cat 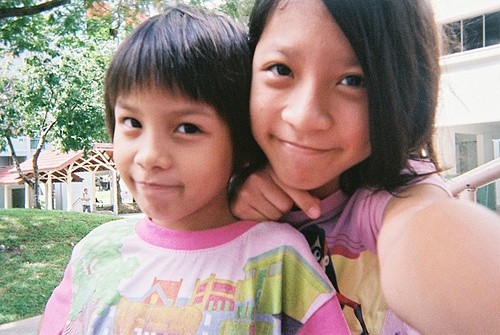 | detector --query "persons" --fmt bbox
[81,188,92,212]
[36,8,351,335]
[229,0,500,334]
